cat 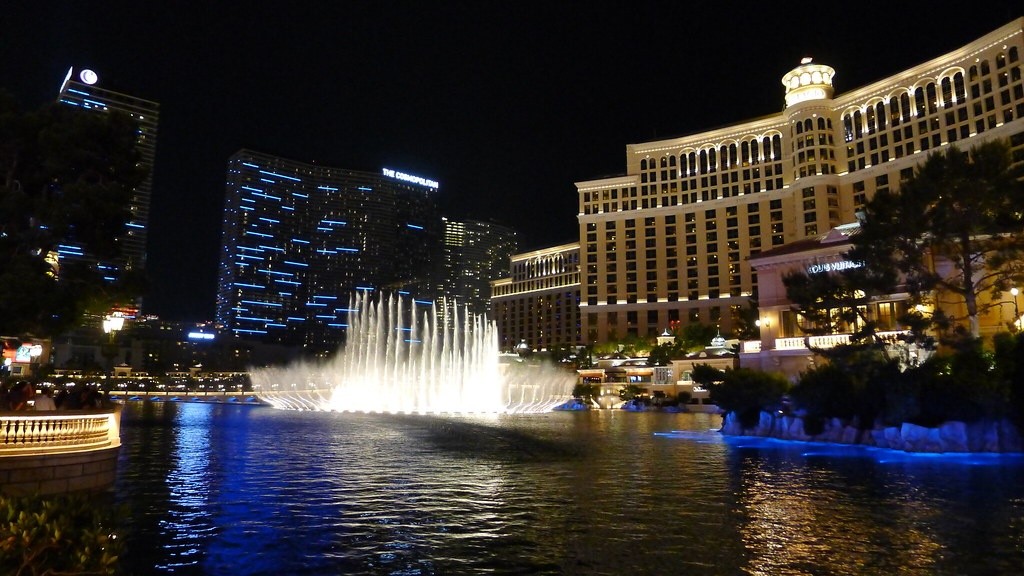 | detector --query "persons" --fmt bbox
[7,379,56,411]
